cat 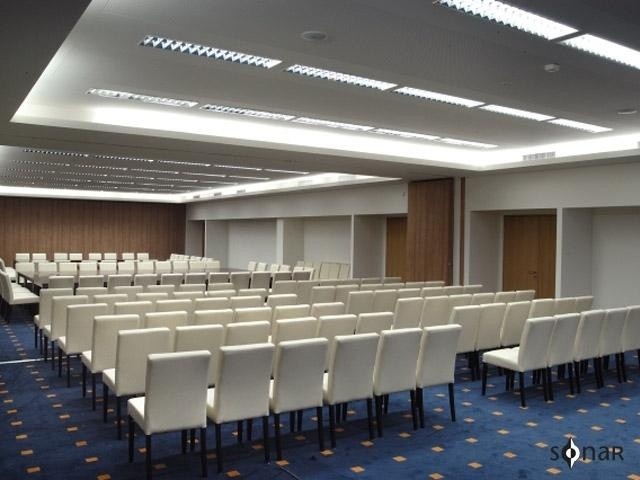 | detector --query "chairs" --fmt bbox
[32,273,640,479]
[0,249,318,324]
